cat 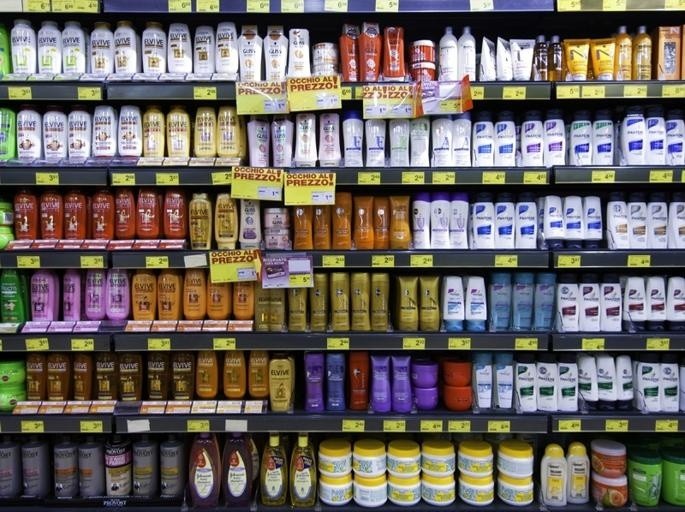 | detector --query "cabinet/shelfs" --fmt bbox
[0,1,684,512]
